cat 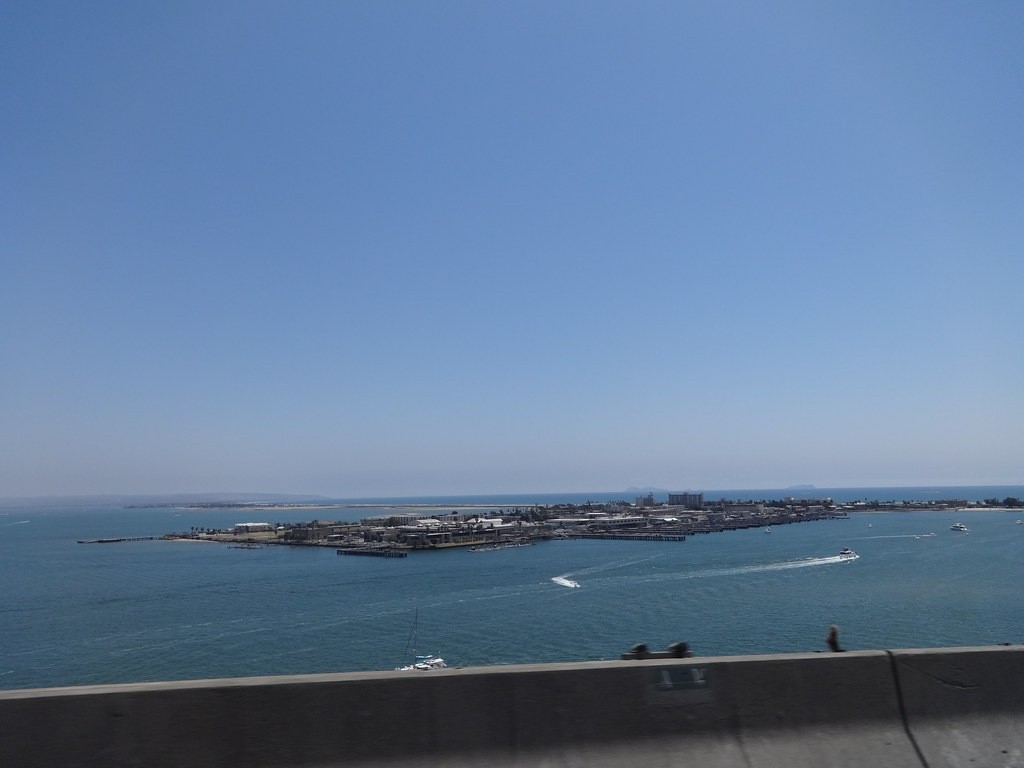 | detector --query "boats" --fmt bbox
[840,549,856,559]
[950,521,969,532]
